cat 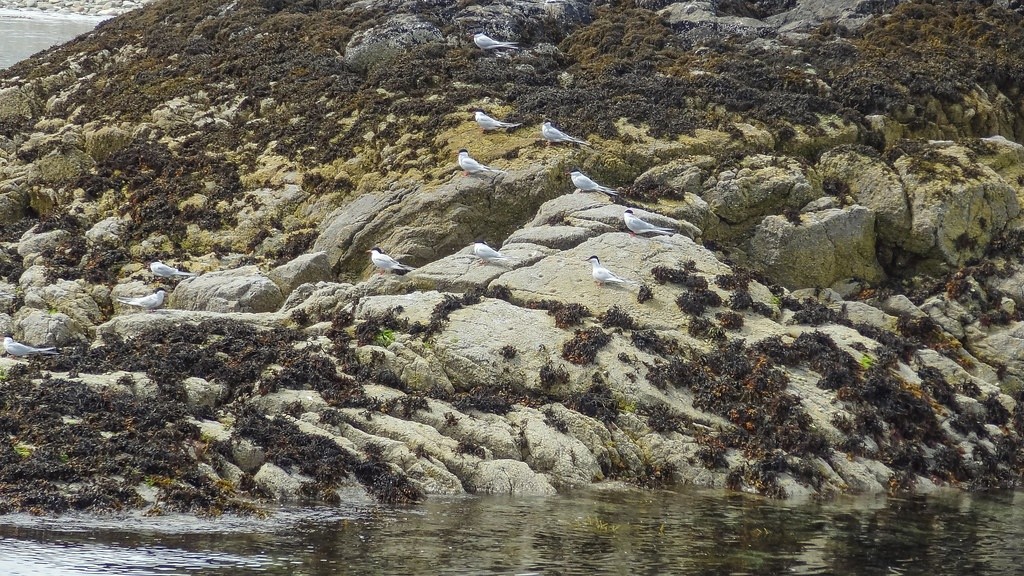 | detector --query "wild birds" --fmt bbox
[471,30,519,53]
[623,210,674,240]
[115,259,196,313]
[0,336,60,359]
[467,239,519,265]
[585,255,626,286]
[458,111,620,196]
[366,246,418,274]
